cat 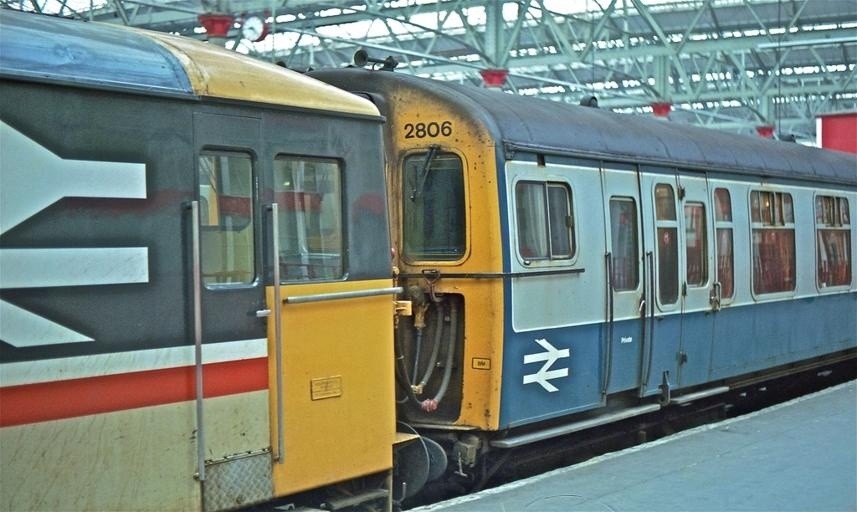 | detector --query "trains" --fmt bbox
[0,11,854,510]
